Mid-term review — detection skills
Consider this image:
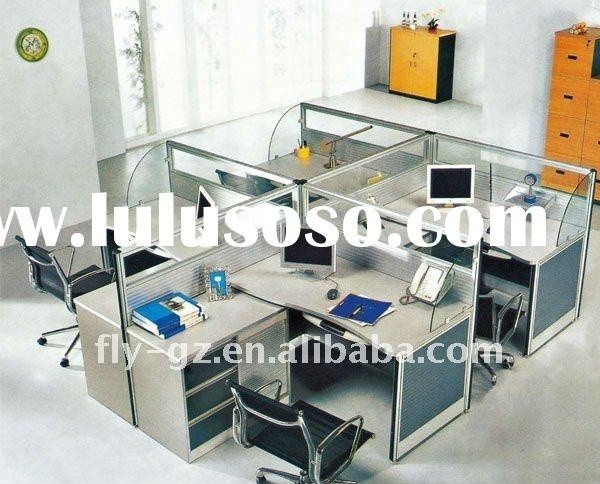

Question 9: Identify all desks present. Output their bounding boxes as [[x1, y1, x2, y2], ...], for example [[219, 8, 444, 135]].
[[72, 102, 596, 464]]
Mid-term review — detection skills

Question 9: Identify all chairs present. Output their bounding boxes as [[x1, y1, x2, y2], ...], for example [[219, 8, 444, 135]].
[[14, 233, 116, 365], [223, 378, 374, 483]]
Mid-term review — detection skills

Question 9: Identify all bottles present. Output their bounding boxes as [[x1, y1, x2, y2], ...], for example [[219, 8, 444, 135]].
[[402, 11, 419, 29]]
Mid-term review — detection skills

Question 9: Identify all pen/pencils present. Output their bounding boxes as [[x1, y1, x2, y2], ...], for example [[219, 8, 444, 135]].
[[349, 306, 363, 317], [297, 138, 306, 148]]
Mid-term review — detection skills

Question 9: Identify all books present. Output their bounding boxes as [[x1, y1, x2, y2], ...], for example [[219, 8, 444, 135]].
[[131, 290, 208, 340]]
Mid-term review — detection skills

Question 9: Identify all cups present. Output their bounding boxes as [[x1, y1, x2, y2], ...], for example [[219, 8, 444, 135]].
[[295, 146, 312, 159]]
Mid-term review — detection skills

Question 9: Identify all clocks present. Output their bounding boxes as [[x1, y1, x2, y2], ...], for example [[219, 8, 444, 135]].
[[16, 27, 48, 62]]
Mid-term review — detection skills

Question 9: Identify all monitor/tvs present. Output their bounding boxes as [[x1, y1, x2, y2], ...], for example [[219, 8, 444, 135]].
[[426, 164, 475, 213], [195, 184, 216, 231], [279, 221, 337, 281]]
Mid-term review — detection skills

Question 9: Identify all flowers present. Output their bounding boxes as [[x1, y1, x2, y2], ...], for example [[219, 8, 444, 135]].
[[422, 8, 447, 25]]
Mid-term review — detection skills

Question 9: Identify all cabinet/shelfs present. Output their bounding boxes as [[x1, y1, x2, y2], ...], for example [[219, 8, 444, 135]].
[[539, 25, 600, 204], [388, 23, 456, 104]]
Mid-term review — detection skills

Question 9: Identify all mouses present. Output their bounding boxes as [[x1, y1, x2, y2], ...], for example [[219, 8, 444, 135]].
[[327, 289, 338, 300]]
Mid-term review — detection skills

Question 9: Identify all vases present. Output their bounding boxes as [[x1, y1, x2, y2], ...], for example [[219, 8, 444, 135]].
[[429, 25, 438, 33]]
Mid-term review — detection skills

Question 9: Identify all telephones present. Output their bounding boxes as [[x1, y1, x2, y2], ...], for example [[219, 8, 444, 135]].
[[409, 262, 448, 306]]
